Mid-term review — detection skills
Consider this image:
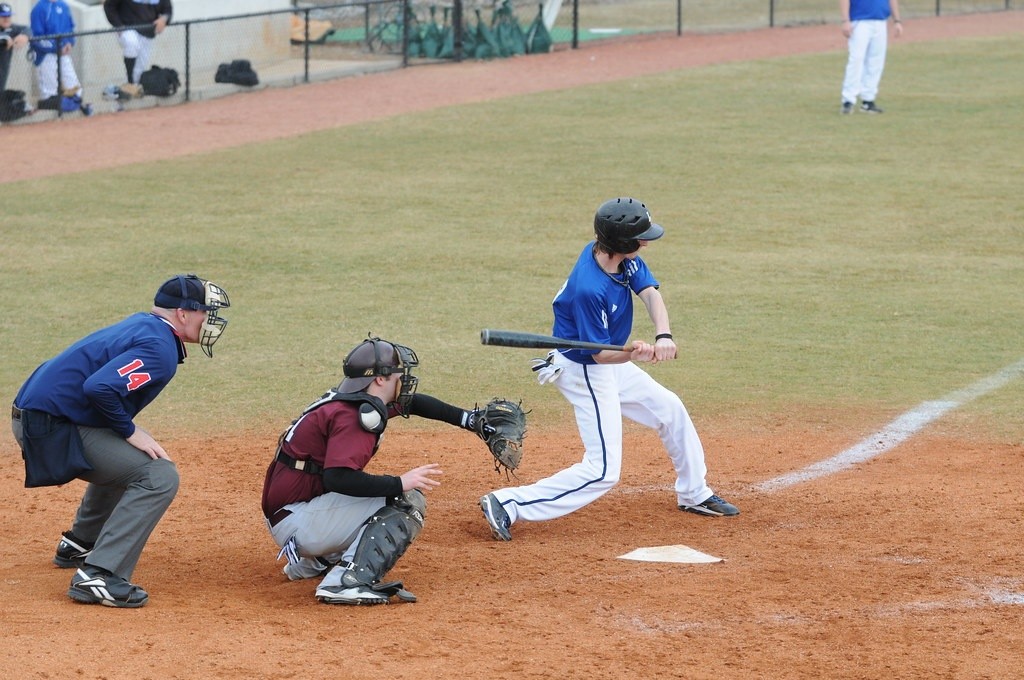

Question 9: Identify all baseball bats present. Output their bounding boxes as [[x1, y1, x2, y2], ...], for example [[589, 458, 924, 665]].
[[480, 328, 679, 359]]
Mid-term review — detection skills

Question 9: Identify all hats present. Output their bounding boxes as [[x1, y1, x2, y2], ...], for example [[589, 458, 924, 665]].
[[0, 3, 12, 16]]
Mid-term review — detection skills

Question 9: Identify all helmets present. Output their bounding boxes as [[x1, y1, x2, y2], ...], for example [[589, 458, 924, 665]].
[[593, 196, 664, 254], [154, 273, 205, 310], [336, 332, 400, 394]]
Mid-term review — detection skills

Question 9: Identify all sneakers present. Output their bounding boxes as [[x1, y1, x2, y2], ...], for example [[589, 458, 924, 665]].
[[68, 564, 149, 608], [52, 530, 94, 569], [677, 494, 740, 518], [314, 586, 390, 606], [840, 102, 854, 114], [859, 101, 882, 114], [477, 494, 514, 543], [283, 562, 300, 582]]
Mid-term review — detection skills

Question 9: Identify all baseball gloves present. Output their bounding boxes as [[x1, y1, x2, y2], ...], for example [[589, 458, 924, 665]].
[[474, 400, 526, 471]]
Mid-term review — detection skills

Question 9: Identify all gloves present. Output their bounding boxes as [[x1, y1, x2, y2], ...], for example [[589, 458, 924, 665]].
[[530, 354, 563, 385]]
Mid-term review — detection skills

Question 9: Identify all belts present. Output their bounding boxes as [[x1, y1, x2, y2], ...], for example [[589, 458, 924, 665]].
[[269, 510, 292, 527], [11, 404, 21, 418]]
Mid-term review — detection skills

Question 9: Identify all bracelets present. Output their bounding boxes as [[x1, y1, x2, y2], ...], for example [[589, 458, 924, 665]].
[[894, 19, 902, 23], [656, 333, 672, 341]]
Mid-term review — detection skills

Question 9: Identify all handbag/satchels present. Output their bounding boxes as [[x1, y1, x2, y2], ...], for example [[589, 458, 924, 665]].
[[140, 66, 180, 96], [214, 59, 259, 87]]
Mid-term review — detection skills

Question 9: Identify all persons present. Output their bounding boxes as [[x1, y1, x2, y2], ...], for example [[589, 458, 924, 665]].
[[0, 4, 33, 92], [27, 1, 83, 102], [104, 0, 172, 84], [12, 274, 230, 606], [262, 335, 528, 605], [480, 199, 740, 541], [839, 0, 904, 114]]
